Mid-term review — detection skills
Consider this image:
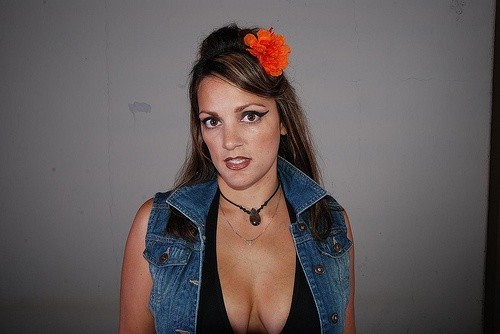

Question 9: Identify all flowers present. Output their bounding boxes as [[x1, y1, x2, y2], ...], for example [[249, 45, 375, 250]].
[[244, 27, 290, 77]]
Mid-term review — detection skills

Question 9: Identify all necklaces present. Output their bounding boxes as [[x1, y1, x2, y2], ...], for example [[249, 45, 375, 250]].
[[217, 179, 281, 227], [216, 189, 283, 248]]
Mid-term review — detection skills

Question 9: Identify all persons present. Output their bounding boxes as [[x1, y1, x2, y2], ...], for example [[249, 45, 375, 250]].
[[117, 23, 359, 334]]
